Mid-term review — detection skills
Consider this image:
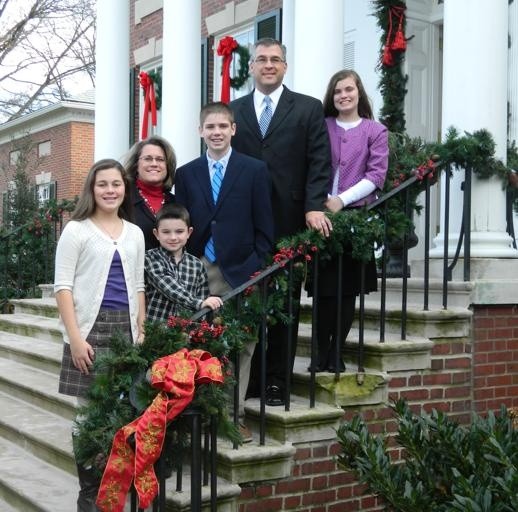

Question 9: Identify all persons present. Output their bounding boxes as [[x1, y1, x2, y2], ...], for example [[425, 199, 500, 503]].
[[173, 101, 276, 444], [54, 157, 148, 511], [303, 69, 391, 374], [119, 135, 184, 253], [227, 37, 336, 409], [144, 204, 226, 479]]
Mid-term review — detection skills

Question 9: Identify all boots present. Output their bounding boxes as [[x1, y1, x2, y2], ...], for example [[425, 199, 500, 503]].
[[72, 430, 102, 512]]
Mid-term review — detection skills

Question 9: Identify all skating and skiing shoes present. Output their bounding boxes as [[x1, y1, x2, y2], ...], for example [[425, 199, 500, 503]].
[[239, 421, 253, 443]]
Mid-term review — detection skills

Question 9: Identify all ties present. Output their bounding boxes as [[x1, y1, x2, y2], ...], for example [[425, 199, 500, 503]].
[[205, 161, 224, 263], [259, 95, 273, 138]]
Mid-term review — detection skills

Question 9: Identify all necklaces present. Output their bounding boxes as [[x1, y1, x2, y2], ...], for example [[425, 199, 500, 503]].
[[95, 215, 120, 240], [138, 190, 166, 218]]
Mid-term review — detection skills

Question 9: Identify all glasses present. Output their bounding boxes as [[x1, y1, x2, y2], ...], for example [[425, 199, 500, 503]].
[[137, 155, 167, 162], [253, 56, 285, 64]]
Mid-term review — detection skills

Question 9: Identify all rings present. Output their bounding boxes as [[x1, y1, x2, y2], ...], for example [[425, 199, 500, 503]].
[[321, 221, 326, 225]]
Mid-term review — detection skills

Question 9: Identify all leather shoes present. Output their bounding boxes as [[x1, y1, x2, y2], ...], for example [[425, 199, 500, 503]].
[[308, 355, 346, 372], [263, 382, 287, 405]]
